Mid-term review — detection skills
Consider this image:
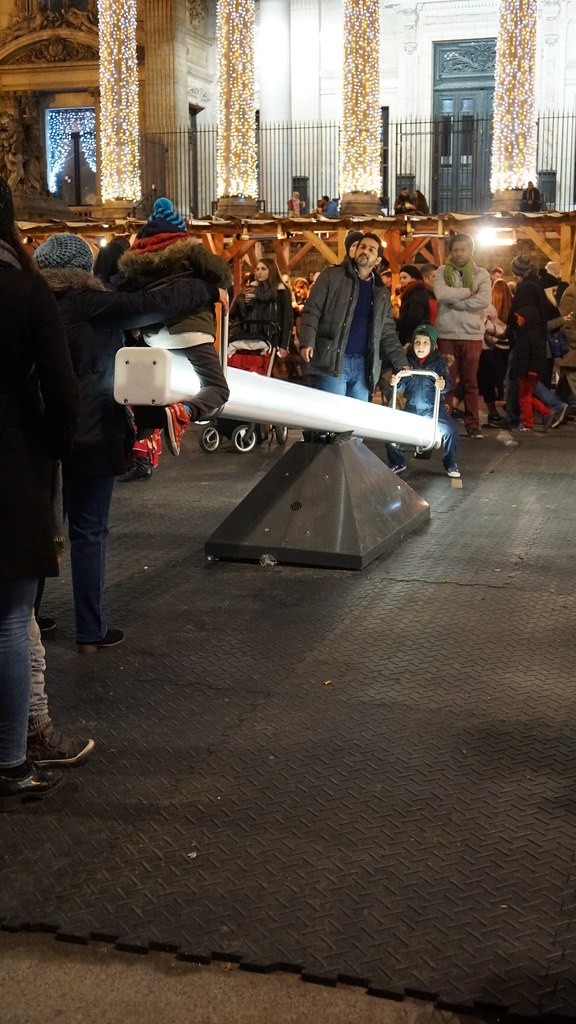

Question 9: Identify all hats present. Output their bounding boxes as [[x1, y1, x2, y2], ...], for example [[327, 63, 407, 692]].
[[344, 229, 365, 253], [33, 233, 93, 272], [148, 197, 186, 231], [413, 320, 437, 346], [137, 221, 184, 238], [399, 264, 423, 279], [511, 254, 532, 278], [545, 262, 562, 279]]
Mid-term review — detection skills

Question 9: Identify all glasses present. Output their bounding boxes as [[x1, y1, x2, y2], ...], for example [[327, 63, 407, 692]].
[[381, 271, 391, 278]]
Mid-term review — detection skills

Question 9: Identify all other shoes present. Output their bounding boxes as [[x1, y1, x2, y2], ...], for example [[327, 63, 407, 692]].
[[551, 403, 569, 428], [512, 424, 534, 432], [488, 415, 504, 424], [496, 429, 518, 446], [543, 412, 554, 431], [468, 428, 483, 438], [450, 409, 464, 418]]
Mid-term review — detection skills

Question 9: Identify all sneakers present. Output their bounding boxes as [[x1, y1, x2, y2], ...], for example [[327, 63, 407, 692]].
[[444, 463, 461, 477], [26, 720, 94, 767], [389, 464, 406, 473], [165, 403, 190, 456]]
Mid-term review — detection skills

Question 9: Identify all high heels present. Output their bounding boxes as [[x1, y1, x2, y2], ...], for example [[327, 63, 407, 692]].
[[76, 629, 125, 653], [35, 617, 56, 631]]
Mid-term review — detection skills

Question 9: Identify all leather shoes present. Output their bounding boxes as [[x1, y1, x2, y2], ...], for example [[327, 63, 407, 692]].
[[0, 760, 65, 813], [113, 458, 151, 483]]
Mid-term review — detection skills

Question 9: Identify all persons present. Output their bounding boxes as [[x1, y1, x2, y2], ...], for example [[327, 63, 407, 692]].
[[520, 181, 541, 212], [394, 187, 429, 215], [296, 228, 576, 476], [320, 196, 339, 217], [288, 191, 307, 215], [231, 258, 321, 381], [0, 181, 234, 810]]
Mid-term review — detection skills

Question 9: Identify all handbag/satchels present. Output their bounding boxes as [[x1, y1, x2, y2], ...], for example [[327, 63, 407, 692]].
[[548, 331, 570, 357]]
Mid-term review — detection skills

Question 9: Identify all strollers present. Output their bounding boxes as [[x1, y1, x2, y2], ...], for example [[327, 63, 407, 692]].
[[200, 318, 289, 454]]
